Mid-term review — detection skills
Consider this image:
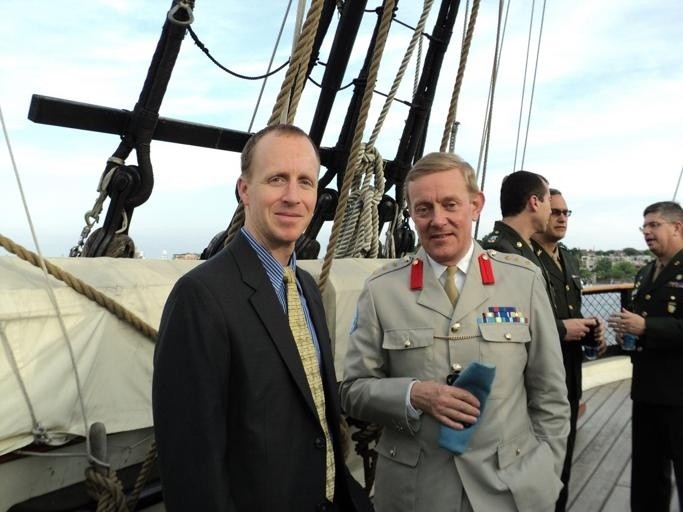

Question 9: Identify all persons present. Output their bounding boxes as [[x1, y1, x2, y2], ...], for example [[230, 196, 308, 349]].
[[526, 186, 609, 510], [476, 166, 576, 510], [336, 152, 574, 511], [147, 121, 374, 511], [604, 201, 683, 511]]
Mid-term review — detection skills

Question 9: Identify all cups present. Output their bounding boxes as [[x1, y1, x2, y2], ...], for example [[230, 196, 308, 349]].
[[583, 317, 601, 360]]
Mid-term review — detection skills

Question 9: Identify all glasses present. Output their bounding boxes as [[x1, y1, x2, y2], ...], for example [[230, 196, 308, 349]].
[[639, 221, 672, 230], [551, 208, 573, 218]]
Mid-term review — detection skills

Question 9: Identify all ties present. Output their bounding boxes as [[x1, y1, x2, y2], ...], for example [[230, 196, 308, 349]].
[[552, 254, 562, 269], [281, 265, 336, 504], [444, 265, 460, 310], [652, 261, 661, 284]]
